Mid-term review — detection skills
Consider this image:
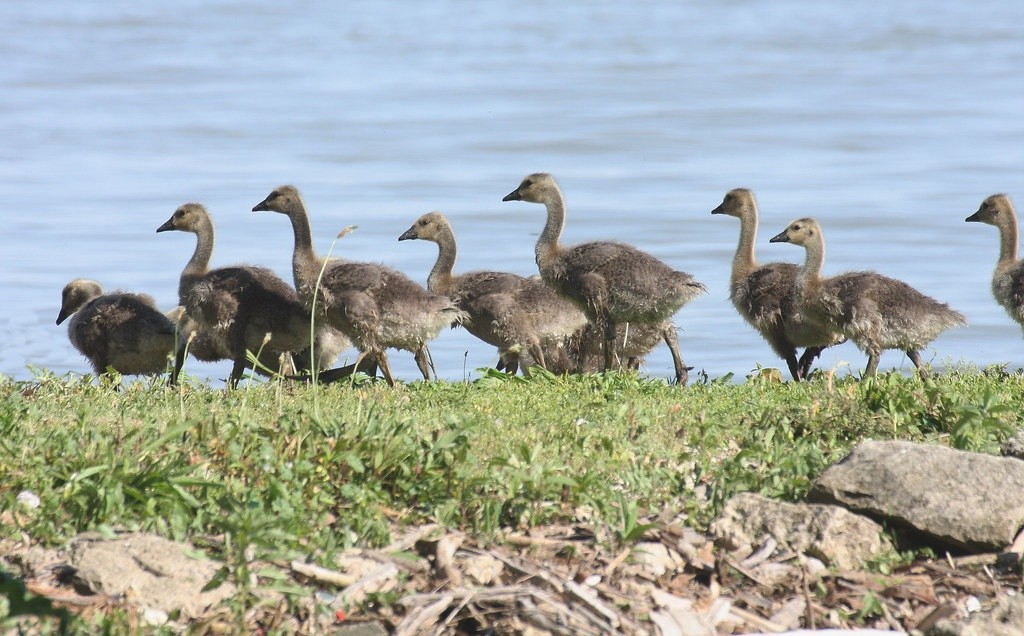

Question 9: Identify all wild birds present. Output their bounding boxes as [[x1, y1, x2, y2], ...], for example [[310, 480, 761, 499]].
[[397, 212, 593, 373], [769, 218, 968, 387], [251, 185, 470, 385], [54, 279, 190, 390], [710, 187, 847, 382], [963, 193, 1024, 327], [519, 275, 647, 379], [161, 303, 351, 380], [503, 171, 710, 387], [156, 204, 326, 390]]
